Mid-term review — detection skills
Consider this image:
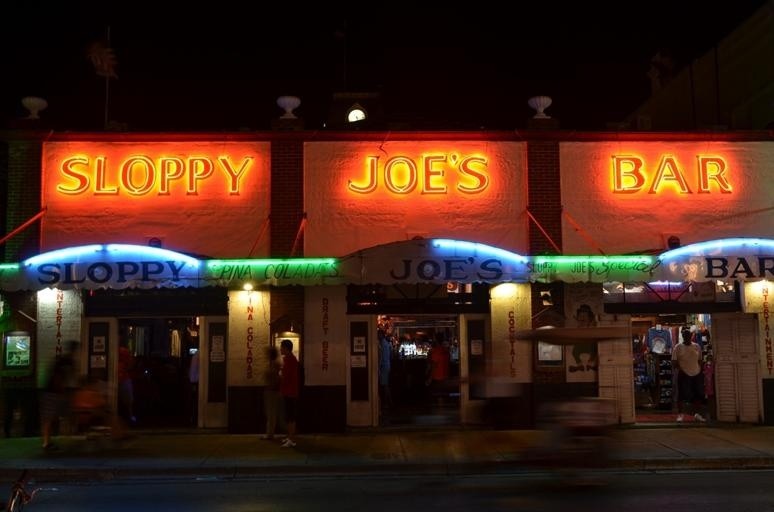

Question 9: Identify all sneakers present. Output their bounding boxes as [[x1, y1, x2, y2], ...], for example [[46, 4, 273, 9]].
[[677, 412, 685, 422], [694, 413, 707, 422], [282, 437, 289, 443], [280, 440, 296, 448]]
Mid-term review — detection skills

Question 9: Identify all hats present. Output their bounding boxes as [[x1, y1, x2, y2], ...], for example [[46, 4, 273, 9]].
[[578, 304, 592, 313]]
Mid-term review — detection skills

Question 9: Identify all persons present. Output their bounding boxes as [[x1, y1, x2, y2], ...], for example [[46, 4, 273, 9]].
[[71, 373, 127, 444], [672, 326, 708, 423], [279, 340, 300, 449], [118, 337, 137, 424], [262, 344, 281, 441], [376, 329, 396, 409], [186, 345, 200, 427], [427, 336, 450, 406], [37, 338, 82, 446]]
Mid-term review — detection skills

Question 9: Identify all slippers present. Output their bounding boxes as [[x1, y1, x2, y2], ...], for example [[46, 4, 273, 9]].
[[41, 441, 58, 451]]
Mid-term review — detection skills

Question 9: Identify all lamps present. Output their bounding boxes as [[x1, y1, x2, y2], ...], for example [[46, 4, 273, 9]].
[[344, 101, 369, 126]]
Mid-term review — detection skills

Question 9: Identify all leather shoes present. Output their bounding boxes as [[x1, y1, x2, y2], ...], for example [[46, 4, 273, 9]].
[[260, 436, 272, 439]]
